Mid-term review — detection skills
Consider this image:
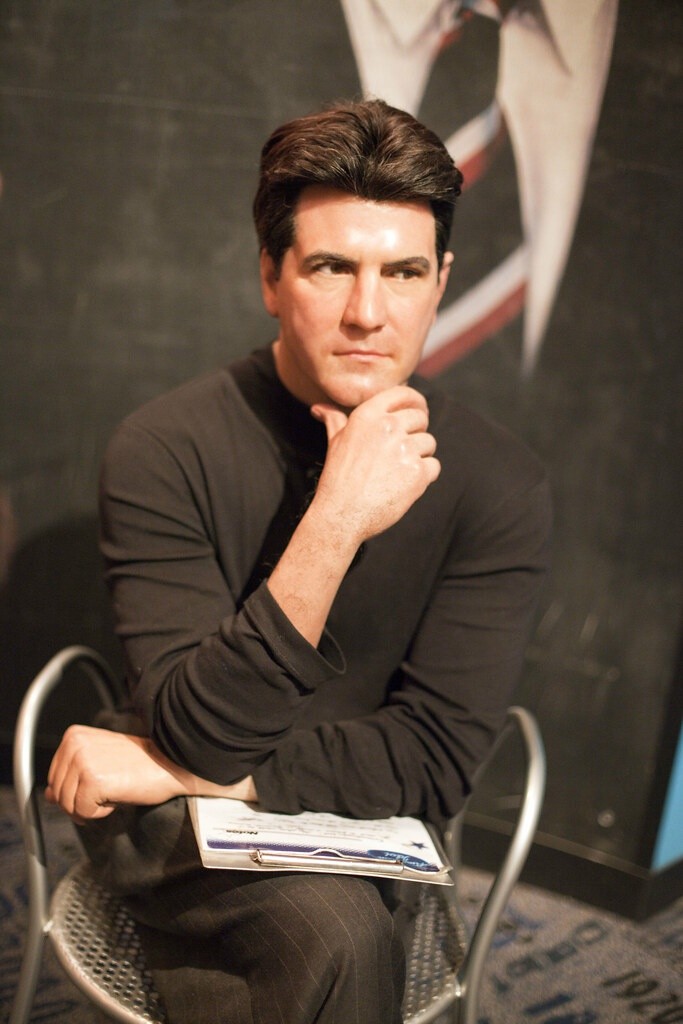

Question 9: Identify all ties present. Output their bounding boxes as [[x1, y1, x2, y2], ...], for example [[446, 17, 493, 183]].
[[412, 0, 526, 422]]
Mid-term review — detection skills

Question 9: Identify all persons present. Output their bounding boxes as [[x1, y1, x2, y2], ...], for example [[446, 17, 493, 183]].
[[183, 0, 682, 843], [43, 103, 549, 1024]]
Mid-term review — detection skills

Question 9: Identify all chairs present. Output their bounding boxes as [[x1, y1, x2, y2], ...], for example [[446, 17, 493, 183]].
[[11, 645, 547, 1024]]
